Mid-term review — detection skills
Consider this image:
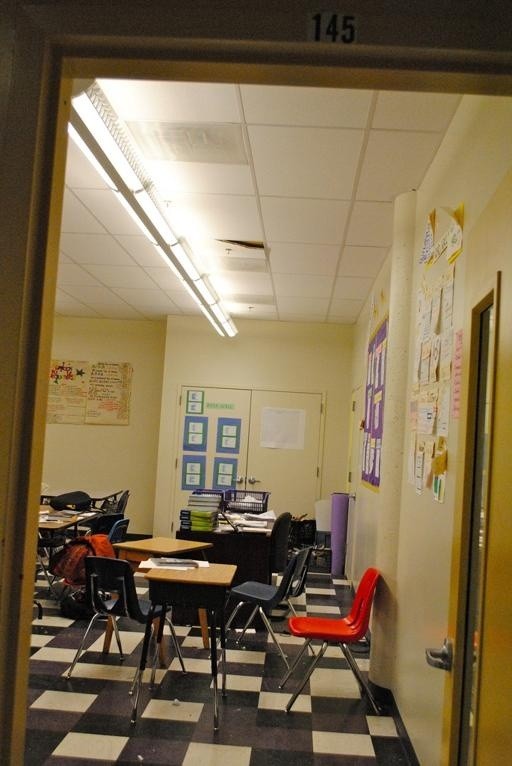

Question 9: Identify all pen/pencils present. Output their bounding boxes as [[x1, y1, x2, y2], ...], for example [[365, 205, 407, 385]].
[[46, 520, 58, 521]]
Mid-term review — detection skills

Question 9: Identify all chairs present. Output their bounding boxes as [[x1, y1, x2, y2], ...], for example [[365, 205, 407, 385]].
[[38, 489, 130, 618], [270, 511, 292, 572], [65, 556, 187, 695], [208, 545, 382, 716]]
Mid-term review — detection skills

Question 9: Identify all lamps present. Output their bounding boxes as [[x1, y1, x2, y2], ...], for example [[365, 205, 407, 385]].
[[67, 90, 238, 339]]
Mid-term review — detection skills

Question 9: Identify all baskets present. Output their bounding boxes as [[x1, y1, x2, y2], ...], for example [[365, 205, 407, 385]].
[[223, 488, 272, 515]]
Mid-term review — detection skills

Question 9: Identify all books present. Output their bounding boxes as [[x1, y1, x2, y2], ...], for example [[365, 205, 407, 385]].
[[179, 488, 268, 532], [46, 513, 72, 522], [151, 557, 198, 569]]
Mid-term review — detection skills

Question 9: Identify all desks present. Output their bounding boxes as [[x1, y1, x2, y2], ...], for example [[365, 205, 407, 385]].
[[171, 529, 272, 627], [111, 536, 239, 732]]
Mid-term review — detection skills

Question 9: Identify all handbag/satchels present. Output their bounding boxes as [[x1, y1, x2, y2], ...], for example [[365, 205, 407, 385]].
[[49, 490, 93, 512]]
